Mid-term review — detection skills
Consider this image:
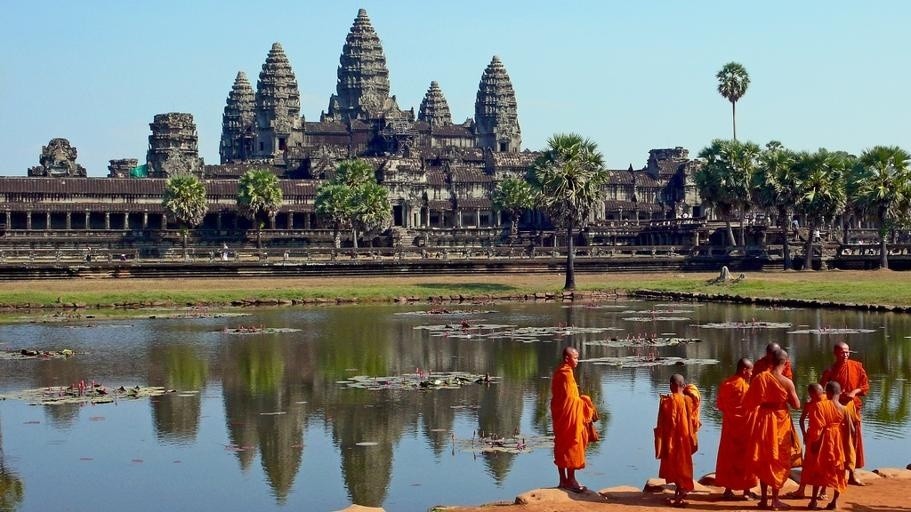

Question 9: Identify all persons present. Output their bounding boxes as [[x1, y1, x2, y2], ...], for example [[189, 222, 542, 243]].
[[858, 239, 863, 255], [814, 228, 821, 242], [550, 346, 600, 494], [653, 342, 870, 510]]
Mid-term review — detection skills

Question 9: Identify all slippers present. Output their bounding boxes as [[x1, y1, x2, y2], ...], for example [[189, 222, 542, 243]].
[[667, 496, 688, 507], [783, 490, 804, 498], [808, 494, 838, 509], [758, 499, 791, 510], [720, 491, 755, 500], [849, 479, 866, 486], [563, 484, 585, 493]]
